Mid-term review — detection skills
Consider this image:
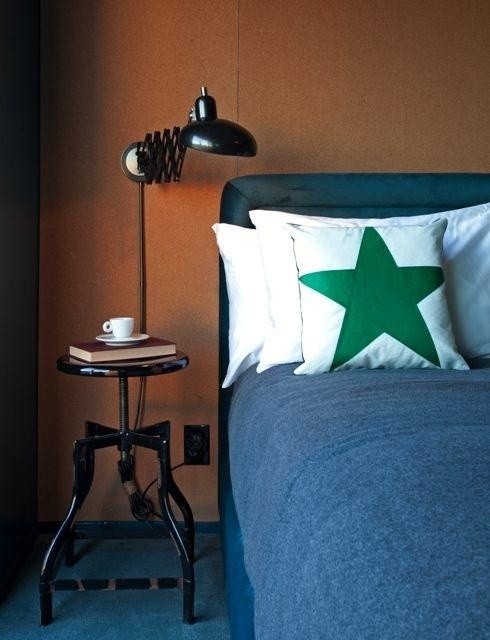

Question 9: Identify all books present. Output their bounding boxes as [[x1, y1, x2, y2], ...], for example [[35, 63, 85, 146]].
[[66, 336, 177, 364]]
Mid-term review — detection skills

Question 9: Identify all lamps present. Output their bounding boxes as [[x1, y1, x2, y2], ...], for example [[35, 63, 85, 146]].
[[115, 84, 258, 523]]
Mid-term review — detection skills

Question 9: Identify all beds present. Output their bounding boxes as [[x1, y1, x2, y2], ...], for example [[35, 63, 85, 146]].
[[218, 171, 490, 640]]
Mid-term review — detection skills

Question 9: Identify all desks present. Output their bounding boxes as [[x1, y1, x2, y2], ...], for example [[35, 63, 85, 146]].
[[36, 352, 199, 627]]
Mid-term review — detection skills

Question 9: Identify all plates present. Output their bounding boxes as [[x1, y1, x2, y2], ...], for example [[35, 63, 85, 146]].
[[96, 332, 150, 347]]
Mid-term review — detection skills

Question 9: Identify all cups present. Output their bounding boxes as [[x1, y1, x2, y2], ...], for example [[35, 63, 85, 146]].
[[102, 316, 135, 339]]
